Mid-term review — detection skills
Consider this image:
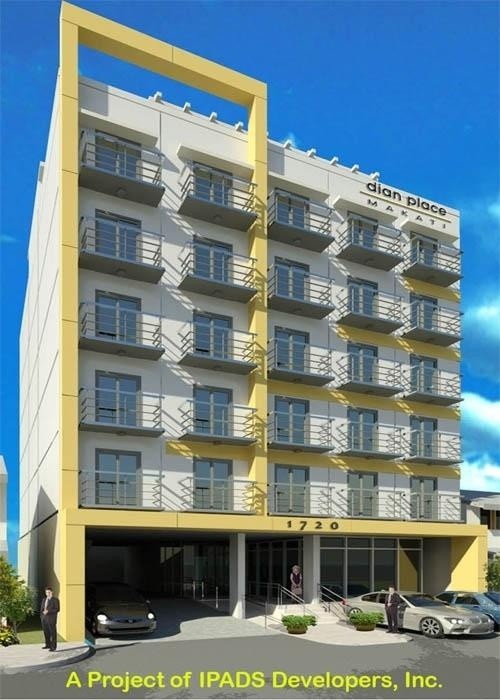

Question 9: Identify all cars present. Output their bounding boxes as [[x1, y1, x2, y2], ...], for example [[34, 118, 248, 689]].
[[342, 591, 500, 638], [85, 584, 158, 638]]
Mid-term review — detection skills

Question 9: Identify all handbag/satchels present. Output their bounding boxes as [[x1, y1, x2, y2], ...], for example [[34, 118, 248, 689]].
[[291, 585, 302, 595]]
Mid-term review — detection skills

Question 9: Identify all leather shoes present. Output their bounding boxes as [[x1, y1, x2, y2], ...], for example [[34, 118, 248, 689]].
[[42, 647, 56, 651]]
